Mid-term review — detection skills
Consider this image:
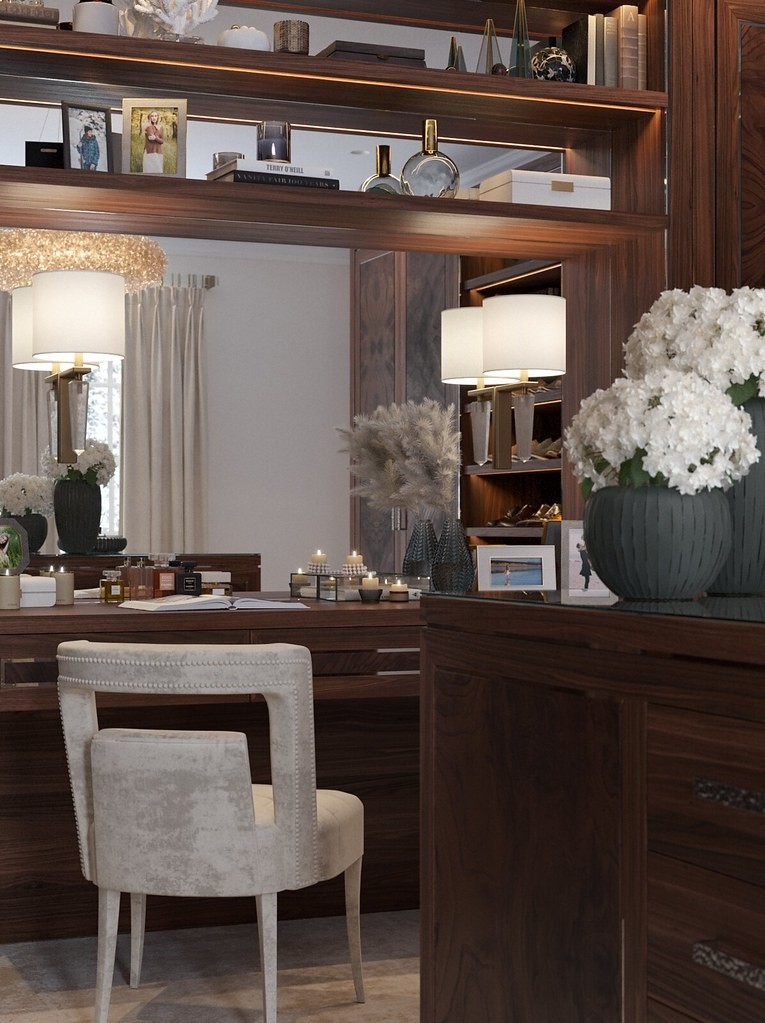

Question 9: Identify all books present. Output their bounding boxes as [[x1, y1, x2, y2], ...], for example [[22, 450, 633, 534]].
[[561, 5, 647, 90], [205, 159, 340, 190], [119, 595, 311, 611], [0, 1, 59, 30]]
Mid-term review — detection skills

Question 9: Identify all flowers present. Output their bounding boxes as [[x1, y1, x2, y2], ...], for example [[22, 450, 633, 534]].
[[563, 367, 761, 497], [0, 472, 54, 518], [621, 284, 765, 406], [0, 437, 117, 519]]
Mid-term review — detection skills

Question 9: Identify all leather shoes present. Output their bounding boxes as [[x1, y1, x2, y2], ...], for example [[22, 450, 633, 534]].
[[524, 503, 561, 524], [515, 504, 551, 526], [486, 505, 523, 527], [499, 504, 539, 527]]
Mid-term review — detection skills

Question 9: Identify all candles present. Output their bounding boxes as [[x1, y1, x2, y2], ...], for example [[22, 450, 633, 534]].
[[391, 583, 408, 591], [362, 578, 379, 589], [51, 571, 74, 605], [312, 554, 327, 564], [292, 574, 308, 583], [347, 555, 363, 564], [43, 572, 51, 577], [0, 576, 21, 610]]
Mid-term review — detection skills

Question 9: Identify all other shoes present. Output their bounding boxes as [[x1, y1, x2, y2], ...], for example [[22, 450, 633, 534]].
[[513, 377, 561, 394], [487, 437, 561, 460]]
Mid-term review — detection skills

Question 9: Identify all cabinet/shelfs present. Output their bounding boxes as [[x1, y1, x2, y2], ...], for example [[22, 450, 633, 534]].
[[420, 597, 765, 1023], [457, 260, 571, 604], [0, 0, 670, 254]]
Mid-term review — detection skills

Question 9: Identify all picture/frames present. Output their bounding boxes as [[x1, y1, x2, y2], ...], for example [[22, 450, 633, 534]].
[[0, 516, 30, 576], [475, 545, 557, 591], [118, 98, 187, 178], [62, 100, 114, 172], [560, 519, 618, 606]]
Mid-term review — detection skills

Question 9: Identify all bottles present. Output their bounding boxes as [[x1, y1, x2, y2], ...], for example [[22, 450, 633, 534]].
[[116, 557, 136, 600], [100, 571, 124, 602], [130, 557, 153, 600], [359, 145, 401, 195], [400, 119, 460, 199], [177, 562, 201, 596], [147, 553, 179, 598]]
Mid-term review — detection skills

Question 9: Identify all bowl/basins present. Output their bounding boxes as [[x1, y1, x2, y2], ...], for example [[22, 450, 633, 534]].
[[218, 29, 271, 51]]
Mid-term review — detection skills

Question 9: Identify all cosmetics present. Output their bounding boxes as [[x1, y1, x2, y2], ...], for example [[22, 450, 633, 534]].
[[360, 118, 460, 199], [213, 152, 244, 169], [99, 554, 201, 602], [256, 121, 292, 163]]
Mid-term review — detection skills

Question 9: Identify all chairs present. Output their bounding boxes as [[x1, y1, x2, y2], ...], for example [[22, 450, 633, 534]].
[[56, 640, 365, 1023]]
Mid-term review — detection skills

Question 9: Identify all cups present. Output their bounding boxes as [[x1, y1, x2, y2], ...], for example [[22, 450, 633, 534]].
[[257, 120, 291, 163], [73, 2, 119, 36], [213, 152, 245, 170]]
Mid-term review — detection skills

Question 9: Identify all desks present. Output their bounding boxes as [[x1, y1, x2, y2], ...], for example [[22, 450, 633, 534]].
[[0, 590, 422, 943]]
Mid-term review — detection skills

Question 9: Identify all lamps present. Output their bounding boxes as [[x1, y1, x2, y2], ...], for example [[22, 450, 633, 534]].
[[11, 269, 125, 464], [441, 295, 567, 467]]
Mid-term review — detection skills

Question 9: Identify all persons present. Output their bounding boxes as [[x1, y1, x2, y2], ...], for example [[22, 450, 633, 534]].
[[505, 565, 510, 585], [77, 126, 100, 170], [0, 534, 10, 561], [576, 536, 595, 590], [143, 111, 164, 173]]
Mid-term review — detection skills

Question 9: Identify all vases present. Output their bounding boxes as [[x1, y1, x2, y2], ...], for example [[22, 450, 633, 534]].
[[9, 514, 48, 553], [703, 396, 765, 622], [53, 478, 101, 553], [403, 521, 438, 577], [431, 520, 474, 596], [585, 485, 731, 617]]
